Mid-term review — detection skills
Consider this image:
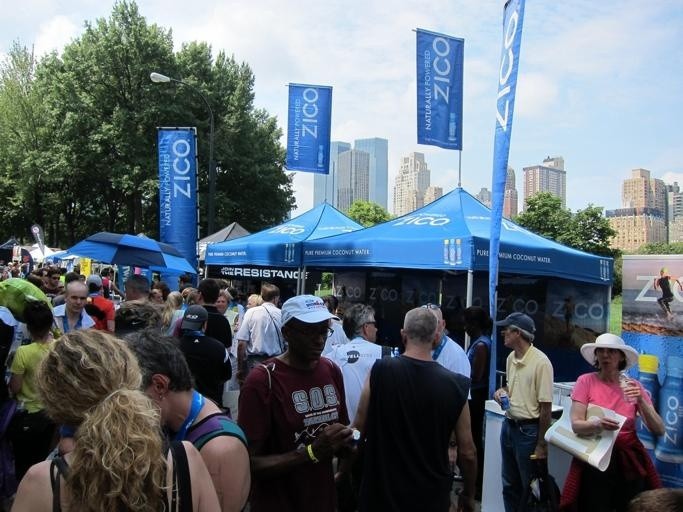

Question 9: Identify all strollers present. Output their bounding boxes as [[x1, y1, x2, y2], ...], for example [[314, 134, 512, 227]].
[[0, 399, 17, 509]]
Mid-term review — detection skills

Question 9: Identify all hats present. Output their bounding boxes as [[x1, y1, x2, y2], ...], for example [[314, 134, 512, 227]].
[[496, 312, 536, 333], [580, 333, 639, 370], [281, 295, 340, 327], [87, 275, 102, 287], [181, 305, 208, 331]]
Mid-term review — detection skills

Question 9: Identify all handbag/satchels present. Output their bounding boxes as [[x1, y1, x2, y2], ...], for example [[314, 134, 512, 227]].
[[527, 459, 561, 512]]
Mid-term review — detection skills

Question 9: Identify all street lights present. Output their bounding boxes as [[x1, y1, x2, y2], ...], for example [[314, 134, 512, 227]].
[[148, 66, 216, 241]]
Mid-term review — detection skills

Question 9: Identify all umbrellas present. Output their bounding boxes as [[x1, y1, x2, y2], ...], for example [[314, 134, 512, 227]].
[[66, 231, 197, 275]]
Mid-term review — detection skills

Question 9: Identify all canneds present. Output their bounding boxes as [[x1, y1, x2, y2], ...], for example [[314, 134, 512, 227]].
[[500, 395, 509, 411]]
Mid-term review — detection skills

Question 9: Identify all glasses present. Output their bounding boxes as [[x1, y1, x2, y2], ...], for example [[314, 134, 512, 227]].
[[291, 325, 334, 337], [421, 304, 438, 309]]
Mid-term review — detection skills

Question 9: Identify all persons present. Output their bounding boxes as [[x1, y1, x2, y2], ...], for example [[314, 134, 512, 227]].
[[630, 487, 683, 512], [494, 312, 555, 512], [559, 332, 665, 512], [654, 269, 683, 320]]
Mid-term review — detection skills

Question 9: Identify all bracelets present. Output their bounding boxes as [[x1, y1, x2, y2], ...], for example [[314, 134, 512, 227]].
[[588, 415, 603, 435]]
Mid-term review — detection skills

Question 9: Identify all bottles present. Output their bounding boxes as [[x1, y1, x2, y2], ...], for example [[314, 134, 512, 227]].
[[500, 396, 510, 411], [393, 347, 399, 356], [631, 354, 662, 450], [617, 370, 637, 405], [657, 356, 682, 463]]
